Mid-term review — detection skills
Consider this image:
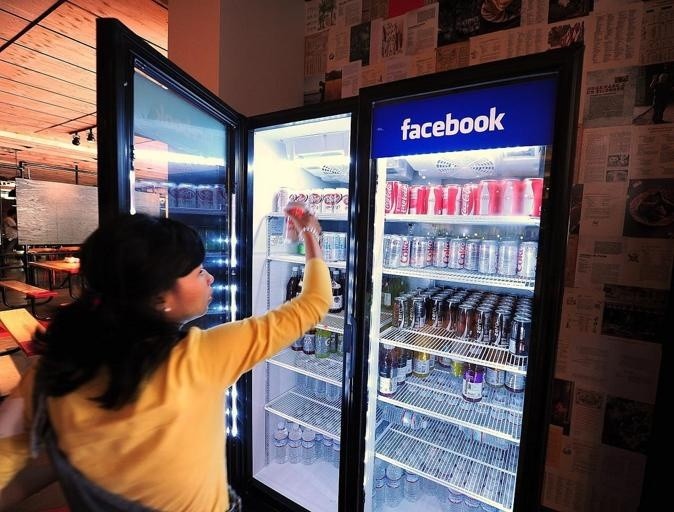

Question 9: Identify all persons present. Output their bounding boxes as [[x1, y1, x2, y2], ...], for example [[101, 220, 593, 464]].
[[3, 206, 18, 251], [0, 208, 333, 512], [650, 73, 671, 124]]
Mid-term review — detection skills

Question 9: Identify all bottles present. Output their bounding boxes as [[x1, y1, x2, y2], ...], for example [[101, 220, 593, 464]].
[[273, 264, 526, 511]]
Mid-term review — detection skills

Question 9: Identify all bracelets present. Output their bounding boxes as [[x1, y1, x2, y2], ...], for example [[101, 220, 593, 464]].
[[300, 227, 319, 242]]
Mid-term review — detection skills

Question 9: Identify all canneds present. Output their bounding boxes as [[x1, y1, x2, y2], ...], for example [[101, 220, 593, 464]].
[[278, 185, 349, 355], [382, 179, 544, 358], [134, 179, 226, 210], [186, 226, 226, 254]]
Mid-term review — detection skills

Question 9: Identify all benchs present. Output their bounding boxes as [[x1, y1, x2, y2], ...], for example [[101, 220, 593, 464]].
[[0, 279, 59, 358]]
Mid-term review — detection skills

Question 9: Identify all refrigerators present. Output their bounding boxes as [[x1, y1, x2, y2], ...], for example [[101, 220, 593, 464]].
[[89, 15, 588, 512]]
